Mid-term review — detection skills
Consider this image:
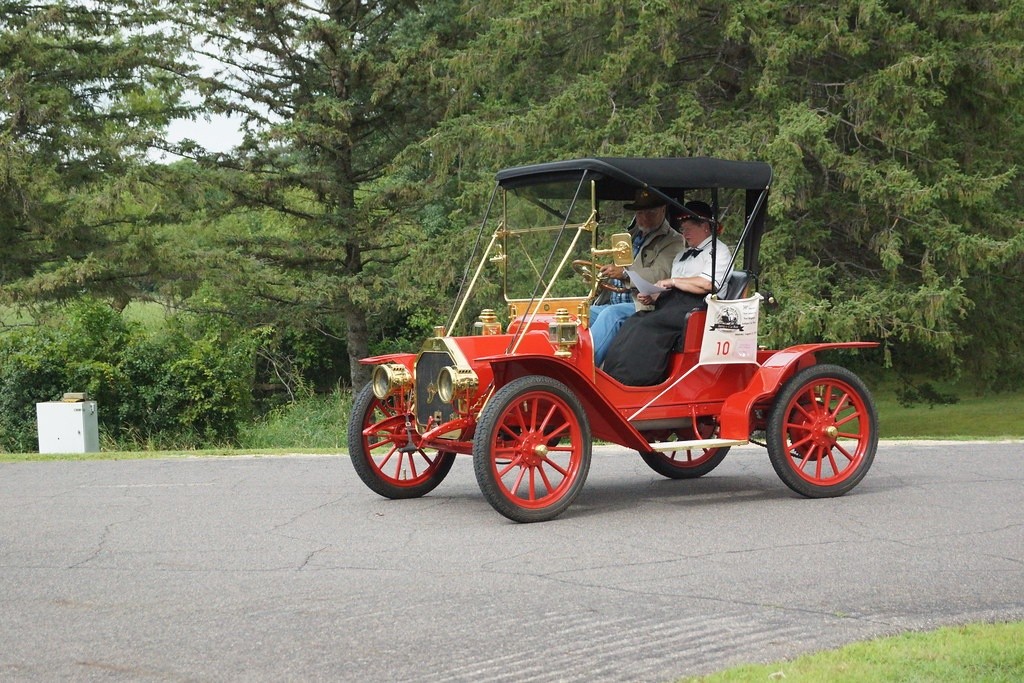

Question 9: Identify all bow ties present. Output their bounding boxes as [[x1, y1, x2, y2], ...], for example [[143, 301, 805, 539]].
[[679, 248, 703, 261]]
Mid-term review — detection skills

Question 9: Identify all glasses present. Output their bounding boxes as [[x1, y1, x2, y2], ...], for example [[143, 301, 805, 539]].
[[635, 207, 663, 216], [679, 223, 701, 232]]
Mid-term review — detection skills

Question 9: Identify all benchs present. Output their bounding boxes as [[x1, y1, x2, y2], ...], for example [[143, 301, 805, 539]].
[[675, 272, 748, 353]]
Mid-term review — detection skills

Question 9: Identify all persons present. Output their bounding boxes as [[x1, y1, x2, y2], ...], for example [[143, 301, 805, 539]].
[[602, 201, 735, 386], [587, 191, 685, 368]]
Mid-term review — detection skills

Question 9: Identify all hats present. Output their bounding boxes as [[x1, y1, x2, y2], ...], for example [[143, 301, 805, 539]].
[[664, 201, 725, 236], [623, 186, 671, 210]]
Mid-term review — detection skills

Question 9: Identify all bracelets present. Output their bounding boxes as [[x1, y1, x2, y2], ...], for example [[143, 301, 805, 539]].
[[622, 270, 628, 280], [672, 277, 676, 287]]
